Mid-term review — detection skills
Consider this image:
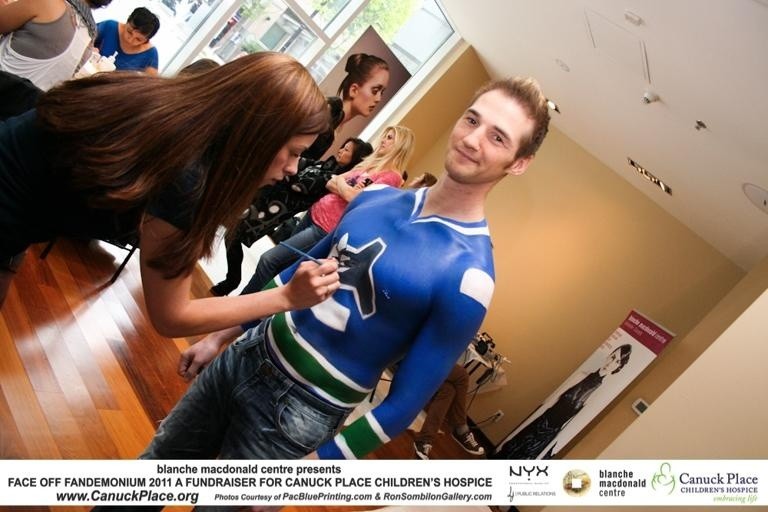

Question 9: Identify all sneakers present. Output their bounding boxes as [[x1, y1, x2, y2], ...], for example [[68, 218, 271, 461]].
[[413, 442, 432, 461], [450, 429, 484, 456]]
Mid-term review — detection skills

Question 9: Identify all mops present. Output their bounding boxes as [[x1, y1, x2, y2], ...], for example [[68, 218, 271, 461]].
[[98, 48, 118, 74]]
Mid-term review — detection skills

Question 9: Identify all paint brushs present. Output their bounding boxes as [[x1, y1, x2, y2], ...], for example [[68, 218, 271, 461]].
[[280, 242, 323, 265]]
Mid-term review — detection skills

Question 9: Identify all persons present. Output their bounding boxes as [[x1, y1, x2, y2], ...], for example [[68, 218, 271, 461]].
[[205, 137, 374, 297], [0, 0, 112, 122], [298, 53, 391, 160], [407, 171, 438, 190], [0, 51, 340, 339], [96, 75, 552, 512], [489, 343, 632, 460], [412, 363, 485, 461], [238, 125, 415, 302], [93, 6, 160, 76]]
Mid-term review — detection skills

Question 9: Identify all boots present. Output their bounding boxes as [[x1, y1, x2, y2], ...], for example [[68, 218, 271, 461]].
[[210, 276, 240, 297]]
